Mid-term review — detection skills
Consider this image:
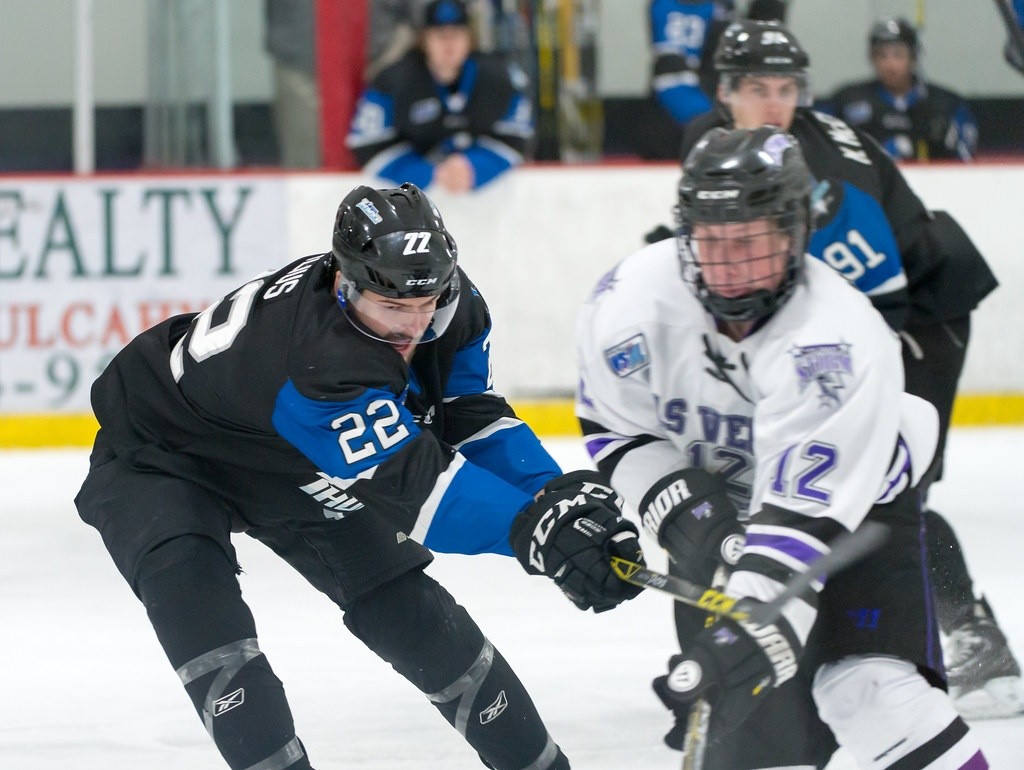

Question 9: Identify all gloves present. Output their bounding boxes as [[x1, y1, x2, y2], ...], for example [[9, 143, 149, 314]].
[[511, 470, 651, 611], [654, 597, 806, 752], [636, 470, 746, 593]]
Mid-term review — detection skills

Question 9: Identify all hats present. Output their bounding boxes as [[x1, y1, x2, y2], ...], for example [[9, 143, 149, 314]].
[[420, 0, 471, 26]]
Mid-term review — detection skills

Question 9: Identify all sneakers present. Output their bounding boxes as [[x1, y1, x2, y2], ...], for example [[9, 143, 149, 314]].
[[939, 595, 1023, 721]]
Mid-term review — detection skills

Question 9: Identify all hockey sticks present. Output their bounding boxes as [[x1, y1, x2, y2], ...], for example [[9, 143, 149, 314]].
[[612, 519, 895, 626], [680, 695, 712, 770]]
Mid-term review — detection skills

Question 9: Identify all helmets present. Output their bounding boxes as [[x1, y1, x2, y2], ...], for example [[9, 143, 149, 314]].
[[866, 17, 919, 63], [675, 125, 815, 324], [716, 19, 816, 107], [336, 181, 461, 344]]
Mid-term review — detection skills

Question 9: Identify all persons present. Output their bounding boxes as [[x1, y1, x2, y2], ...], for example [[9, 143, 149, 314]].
[[676, 18, 1002, 511], [814, 15, 980, 161], [643, 0, 734, 161], [574, 125, 992, 770], [345, 0, 538, 191], [263, 0, 605, 170], [73, 181, 647, 770]]
